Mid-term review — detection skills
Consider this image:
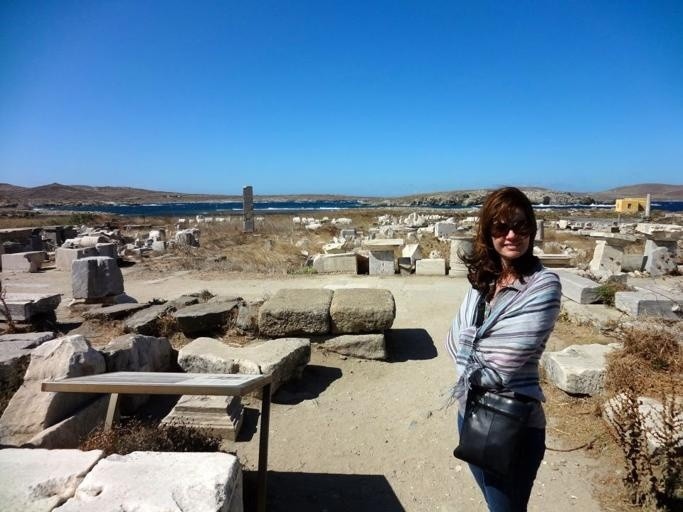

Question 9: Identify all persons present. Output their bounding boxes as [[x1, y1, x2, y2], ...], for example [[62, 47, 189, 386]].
[[611, 222, 620, 233], [446, 187, 561, 512]]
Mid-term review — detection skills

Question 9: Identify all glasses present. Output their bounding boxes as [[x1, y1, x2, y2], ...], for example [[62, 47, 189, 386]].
[[489, 222, 530, 238]]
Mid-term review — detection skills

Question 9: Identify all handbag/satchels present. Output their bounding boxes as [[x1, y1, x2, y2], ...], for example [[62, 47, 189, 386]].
[[453, 385, 530, 475]]
